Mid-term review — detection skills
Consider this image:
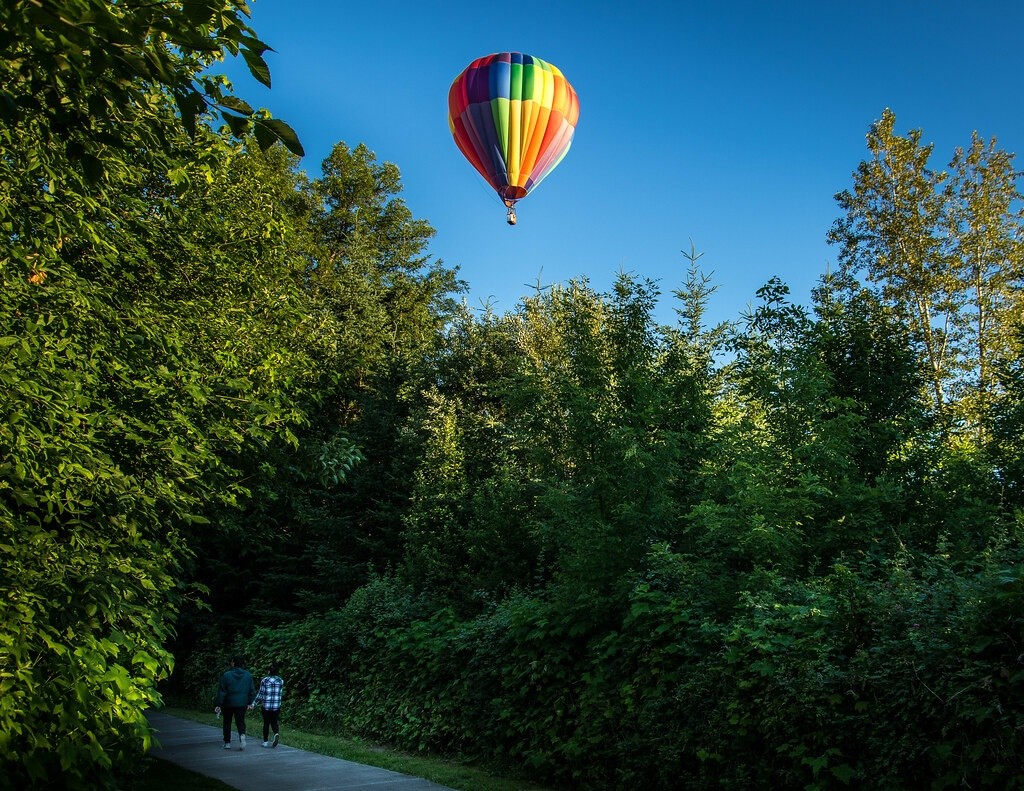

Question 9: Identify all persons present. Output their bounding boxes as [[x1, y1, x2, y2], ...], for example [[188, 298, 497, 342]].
[[250, 665, 284, 748], [213, 656, 256, 750]]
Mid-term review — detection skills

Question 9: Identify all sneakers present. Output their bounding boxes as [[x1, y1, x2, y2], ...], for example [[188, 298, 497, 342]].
[[240, 734, 246, 749], [273, 733, 280, 747], [262, 742, 268, 747], [223, 743, 231, 748]]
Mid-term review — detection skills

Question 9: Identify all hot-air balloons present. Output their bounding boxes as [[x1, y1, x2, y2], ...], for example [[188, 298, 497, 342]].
[[447, 53, 580, 225]]
